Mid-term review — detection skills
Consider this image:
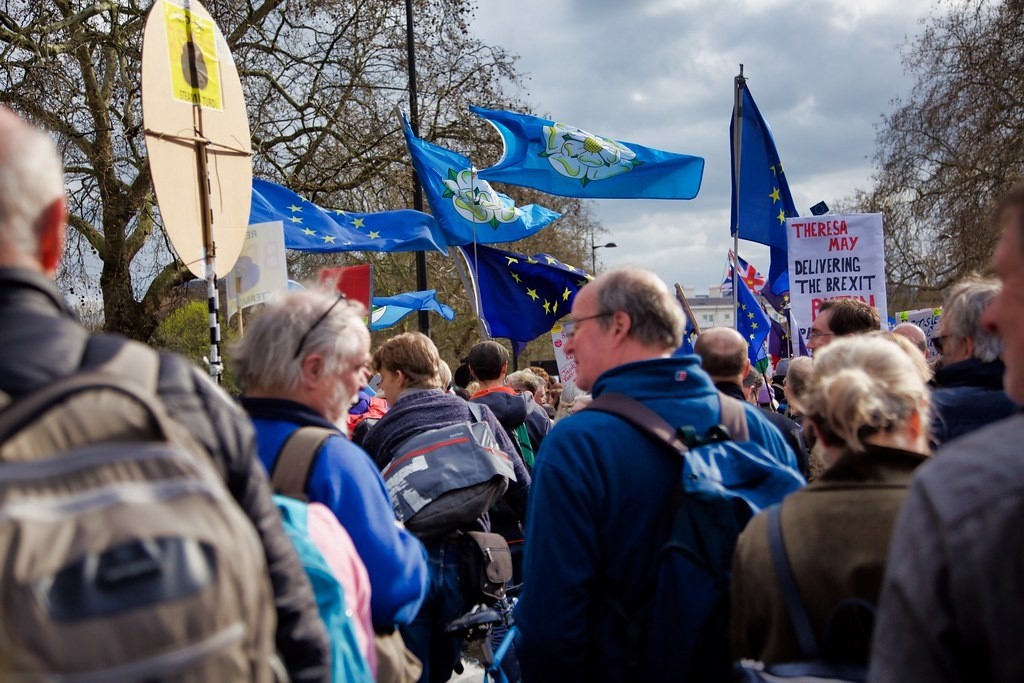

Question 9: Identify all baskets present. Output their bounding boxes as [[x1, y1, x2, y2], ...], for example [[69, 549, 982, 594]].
[[489, 597, 516, 664]]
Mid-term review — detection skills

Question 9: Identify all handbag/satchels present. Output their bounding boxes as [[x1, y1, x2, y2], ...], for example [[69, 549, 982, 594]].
[[382, 420, 517, 530], [466, 531, 515, 602]]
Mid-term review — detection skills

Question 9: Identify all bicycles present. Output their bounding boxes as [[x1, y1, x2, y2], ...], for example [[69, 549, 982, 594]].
[[445, 592, 524, 683]]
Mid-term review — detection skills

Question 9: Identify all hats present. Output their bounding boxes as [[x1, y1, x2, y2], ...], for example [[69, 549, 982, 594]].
[[771, 357, 788, 378], [469, 341, 509, 379], [756, 384, 775, 403]]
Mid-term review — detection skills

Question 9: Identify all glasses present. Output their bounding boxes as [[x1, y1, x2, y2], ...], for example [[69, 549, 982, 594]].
[[561, 310, 612, 337], [294, 290, 347, 359], [806, 330, 837, 340], [932, 333, 954, 354]]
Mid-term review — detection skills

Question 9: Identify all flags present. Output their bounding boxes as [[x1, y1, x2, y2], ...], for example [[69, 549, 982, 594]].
[[721, 84, 809, 366], [458, 242, 595, 341], [470, 105, 705, 200], [674, 289, 696, 345], [249, 179, 449, 257], [403, 128, 564, 246], [370, 290, 455, 330]]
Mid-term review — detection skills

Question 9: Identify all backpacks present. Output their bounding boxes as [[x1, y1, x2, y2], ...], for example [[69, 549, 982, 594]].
[[0, 335, 289, 683], [576, 389, 808, 683], [733, 501, 871, 683]]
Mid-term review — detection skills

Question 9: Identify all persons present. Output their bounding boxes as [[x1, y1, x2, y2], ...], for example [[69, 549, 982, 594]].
[[344, 333, 563, 683], [513, 269, 799, 683], [693, 186, 1024, 683], [0, 106, 331, 683], [228, 288, 432, 638]]
